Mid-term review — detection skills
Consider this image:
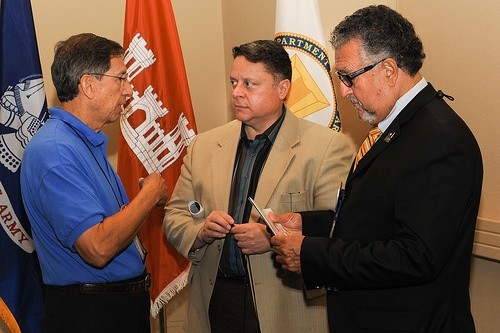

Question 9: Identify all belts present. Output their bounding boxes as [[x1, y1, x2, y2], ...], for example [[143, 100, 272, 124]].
[[60, 272, 152, 292]]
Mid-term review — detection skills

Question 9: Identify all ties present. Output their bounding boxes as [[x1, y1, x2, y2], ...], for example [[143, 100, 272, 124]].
[[353, 128, 383, 172]]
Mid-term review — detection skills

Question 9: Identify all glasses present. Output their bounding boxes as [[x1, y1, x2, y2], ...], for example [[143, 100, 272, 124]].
[[334, 58, 401, 87], [78, 72, 129, 87]]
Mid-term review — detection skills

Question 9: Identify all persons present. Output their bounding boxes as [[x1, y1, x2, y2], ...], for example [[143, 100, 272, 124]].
[[162, 40, 354, 333], [20, 33, 167, 333], [267, 4, 484, 333]]
